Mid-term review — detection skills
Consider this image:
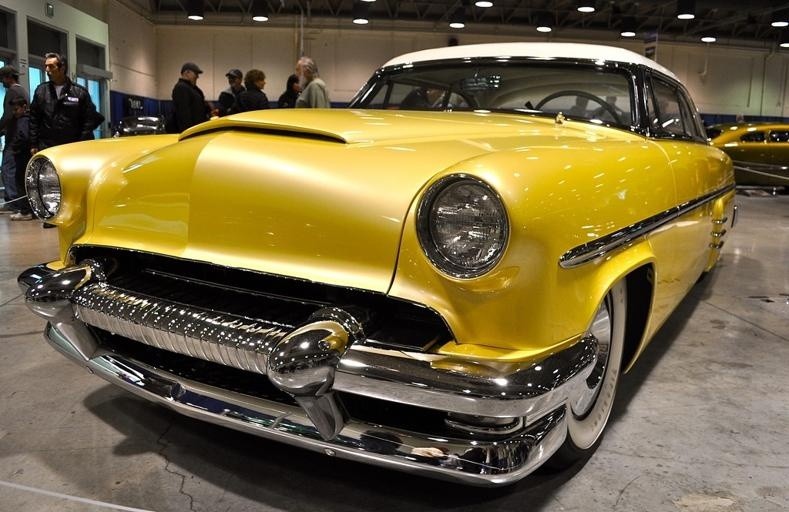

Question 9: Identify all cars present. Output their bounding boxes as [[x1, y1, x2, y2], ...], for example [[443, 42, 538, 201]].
[[16, 40, 740, 491], [110, 116, 165, 136], [700, 119, 789, 190]]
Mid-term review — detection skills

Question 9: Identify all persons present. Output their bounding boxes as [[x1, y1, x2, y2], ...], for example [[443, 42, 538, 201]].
[[0, 64, 31, 211], [9, 97, 34, 220], [219, 69, 246, 117], [294, 56, 330, 108], [399, 86, 445, 110], [171, 62, 212, 132], [236, 69, 270, 111], [29, 53, 96, 228], [278, 74, 302, 108]]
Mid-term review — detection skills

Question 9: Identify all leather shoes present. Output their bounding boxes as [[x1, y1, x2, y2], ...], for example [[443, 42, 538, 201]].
[[42, 222, 56, 228]]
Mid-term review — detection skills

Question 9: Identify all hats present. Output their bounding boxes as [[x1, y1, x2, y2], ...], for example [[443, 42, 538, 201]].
[[180, 62, 203, 75], [225, 68, 242, 80]]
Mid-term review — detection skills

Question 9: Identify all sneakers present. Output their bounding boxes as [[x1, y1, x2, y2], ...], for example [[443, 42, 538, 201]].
[[9, 212, 33, 221]]
